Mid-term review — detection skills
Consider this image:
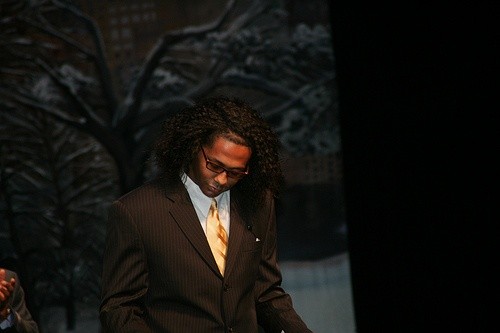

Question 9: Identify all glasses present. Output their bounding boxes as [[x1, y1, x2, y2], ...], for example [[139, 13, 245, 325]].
[[201, 145, 249, 180]]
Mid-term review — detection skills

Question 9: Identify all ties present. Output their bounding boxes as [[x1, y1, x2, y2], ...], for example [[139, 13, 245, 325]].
[[206, 200, 229, 279]]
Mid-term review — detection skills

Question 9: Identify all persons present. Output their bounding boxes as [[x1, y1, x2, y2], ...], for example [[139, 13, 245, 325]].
[[98, 96, 313, 333], [0, 267, 40, 333]]
